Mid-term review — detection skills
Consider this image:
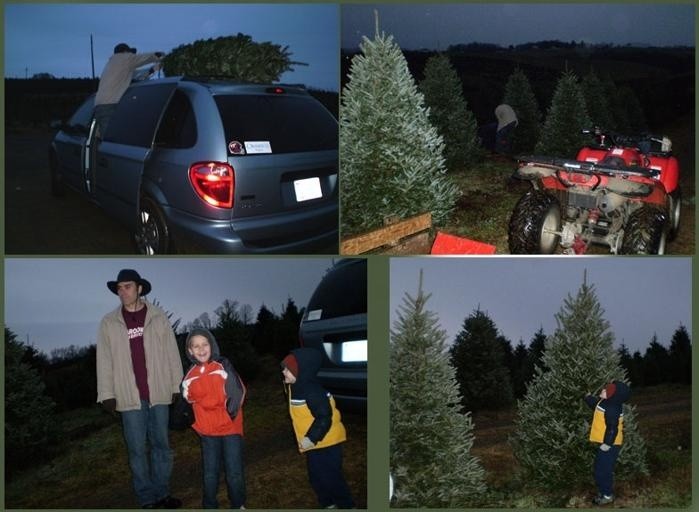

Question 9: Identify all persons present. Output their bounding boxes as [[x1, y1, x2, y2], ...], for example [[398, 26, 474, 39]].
[[281, 347, 355, 509], [179, 327, 247, 508], [92, 43, 165, 141], [581, 380, 631, 506], [494, 103, 519, 156], [95, 269, 185, 509]]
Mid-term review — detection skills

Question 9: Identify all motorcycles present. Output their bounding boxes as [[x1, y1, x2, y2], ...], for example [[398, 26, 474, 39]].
[[502, 127, 684, 255]]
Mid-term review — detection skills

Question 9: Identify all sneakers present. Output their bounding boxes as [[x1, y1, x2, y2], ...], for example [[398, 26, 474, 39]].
[[144, 496, 183, 509], [593, 494, 614, 506]]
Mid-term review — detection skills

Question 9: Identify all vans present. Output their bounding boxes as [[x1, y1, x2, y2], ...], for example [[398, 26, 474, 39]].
[[297, 258, 367, 416], [47, 77, 340, 254]]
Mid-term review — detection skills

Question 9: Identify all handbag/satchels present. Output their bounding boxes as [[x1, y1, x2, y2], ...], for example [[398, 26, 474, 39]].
[[168, 396, 195, 430]]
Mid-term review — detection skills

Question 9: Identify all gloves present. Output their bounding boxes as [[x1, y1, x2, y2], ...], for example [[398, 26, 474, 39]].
[[300, 436, 313, 449], [600, 444, 610, 452], [102, 398, 116, 416]]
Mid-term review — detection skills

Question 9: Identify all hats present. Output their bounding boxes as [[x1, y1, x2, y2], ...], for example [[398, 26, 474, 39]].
[[114, 44, 137, 53], [283, 354, 298, 378], [606, 383, 616, 398], [107, 269, 152, 297]]
